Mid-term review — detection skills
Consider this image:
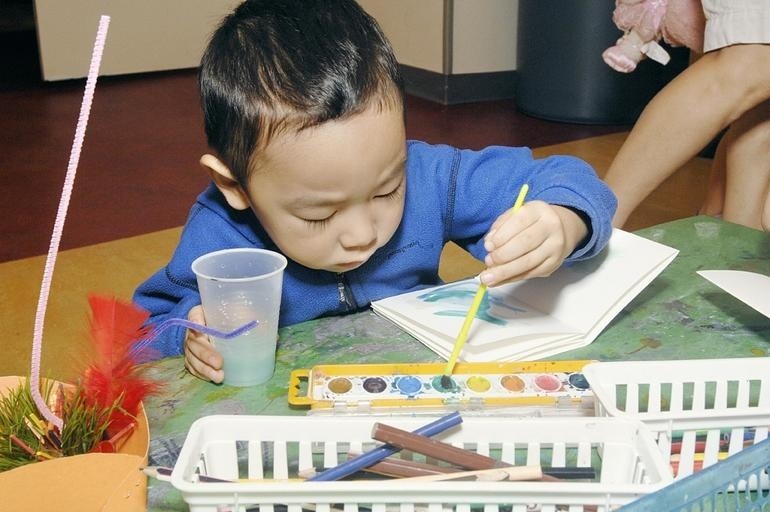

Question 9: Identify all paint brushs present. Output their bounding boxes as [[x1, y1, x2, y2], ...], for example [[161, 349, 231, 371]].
[[441, 183, 529, 388]]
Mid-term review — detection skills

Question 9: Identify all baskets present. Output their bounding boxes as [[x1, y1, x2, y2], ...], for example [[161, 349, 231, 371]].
[[169, 414, 675, 511], [613, 437, 770, 512], [168, 356, 770, 512], [583, 357, 769, 435]]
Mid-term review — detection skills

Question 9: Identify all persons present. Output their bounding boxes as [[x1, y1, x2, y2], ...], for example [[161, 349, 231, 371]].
[[128, 1, 618, 383], [601, 0, 770, 235]]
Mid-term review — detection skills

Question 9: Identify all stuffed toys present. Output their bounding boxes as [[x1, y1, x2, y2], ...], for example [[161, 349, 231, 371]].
[[602, 0, 706, 73]]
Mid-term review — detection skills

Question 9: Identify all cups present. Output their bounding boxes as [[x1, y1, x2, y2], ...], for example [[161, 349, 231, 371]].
[[187, 246, 289, 389]]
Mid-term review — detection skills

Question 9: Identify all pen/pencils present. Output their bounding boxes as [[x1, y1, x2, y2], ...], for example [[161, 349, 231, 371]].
[[656, 427, 770, 479], [305, 411, 595, 483]]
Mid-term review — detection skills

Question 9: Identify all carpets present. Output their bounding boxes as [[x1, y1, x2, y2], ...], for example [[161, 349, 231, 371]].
[[1, 126, 768, 384]]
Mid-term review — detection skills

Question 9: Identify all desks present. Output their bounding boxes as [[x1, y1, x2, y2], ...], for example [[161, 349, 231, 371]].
[[1, 212, 769, 511]]
